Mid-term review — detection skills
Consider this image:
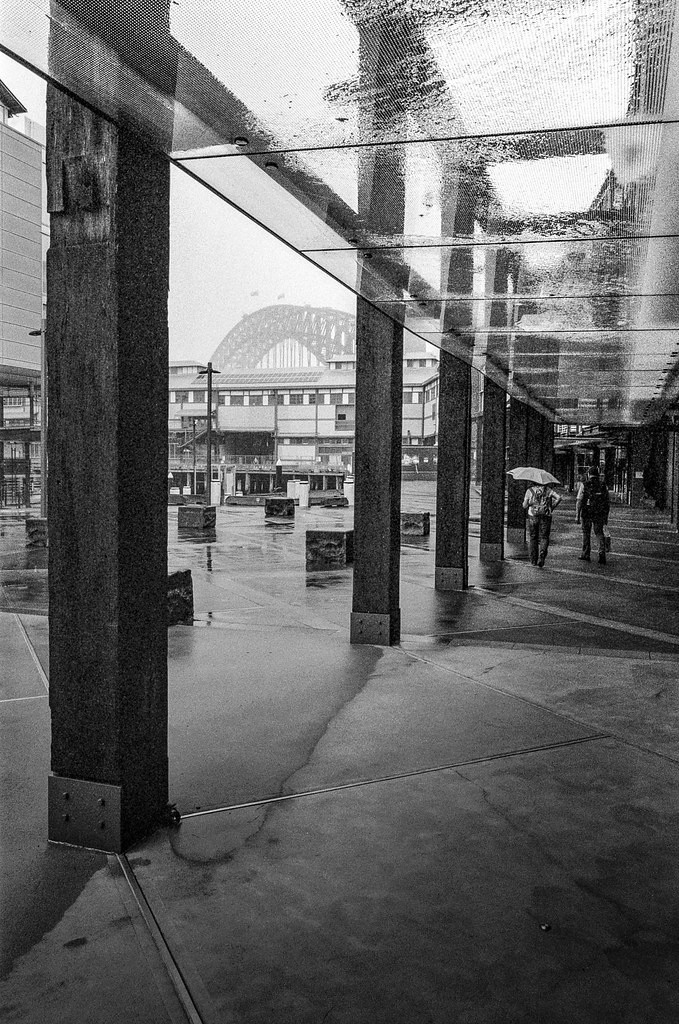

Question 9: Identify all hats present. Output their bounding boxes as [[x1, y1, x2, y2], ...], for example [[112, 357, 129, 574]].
[[586, 467, 597, 473]]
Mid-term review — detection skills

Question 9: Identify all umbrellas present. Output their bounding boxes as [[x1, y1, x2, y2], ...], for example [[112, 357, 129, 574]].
[[506, 466, 561, 485]]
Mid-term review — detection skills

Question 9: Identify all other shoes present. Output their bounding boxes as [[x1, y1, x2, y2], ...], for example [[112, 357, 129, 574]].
[[537, 554, 545, 568], [597, 553, 605, 564], [529, 557, 537, 565], [579, 554, 590, 561]]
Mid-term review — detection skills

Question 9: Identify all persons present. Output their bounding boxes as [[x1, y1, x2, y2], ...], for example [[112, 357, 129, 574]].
[[522, 481, 562, 567], [574, 466, 610, 565]]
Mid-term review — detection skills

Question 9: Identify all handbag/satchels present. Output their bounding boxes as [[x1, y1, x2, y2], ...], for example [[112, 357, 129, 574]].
[[603, 523, 612, 552]]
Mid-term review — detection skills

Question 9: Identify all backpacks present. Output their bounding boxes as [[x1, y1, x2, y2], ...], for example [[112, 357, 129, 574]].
[[587, 481, 609, 518], [529, 486, 549, 516]]
